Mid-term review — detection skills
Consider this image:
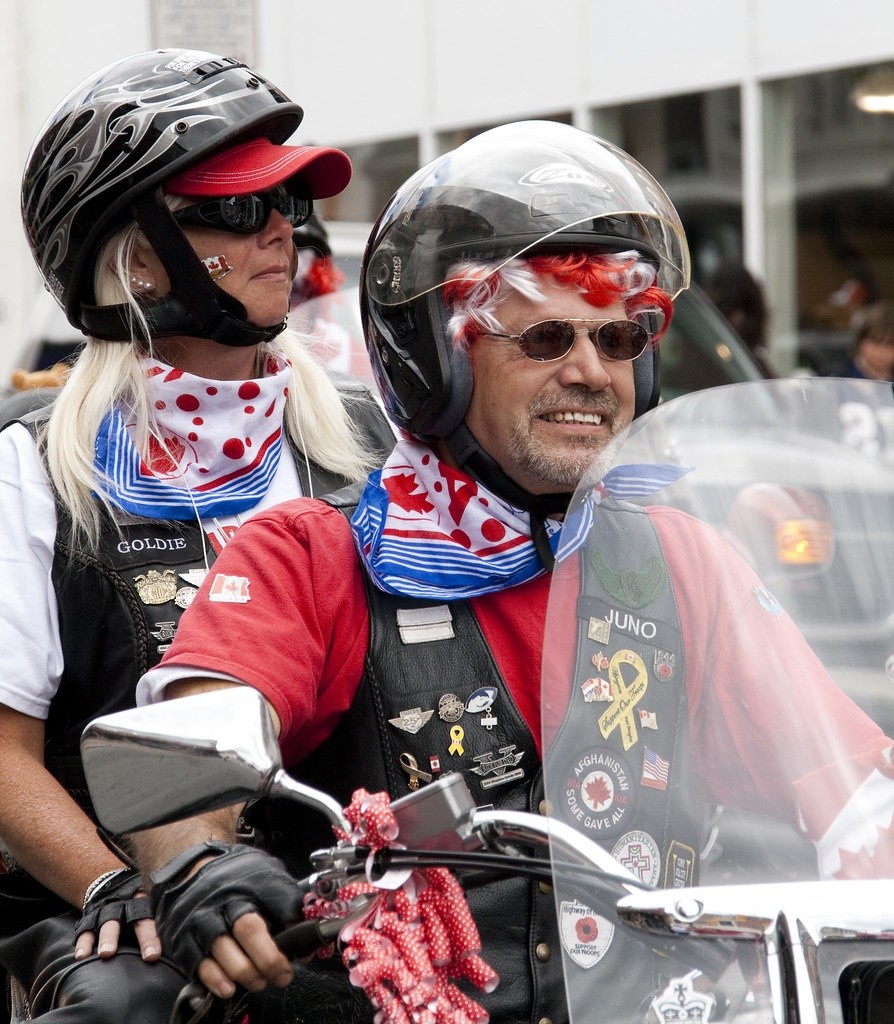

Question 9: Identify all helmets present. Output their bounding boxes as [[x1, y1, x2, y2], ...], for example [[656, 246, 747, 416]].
[[22, 47, 304, 331], [359, 144, 661, 442]]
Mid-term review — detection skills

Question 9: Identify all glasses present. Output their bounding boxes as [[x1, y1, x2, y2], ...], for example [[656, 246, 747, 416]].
[[475, 318, 655, 363], [169, 179, 295, 235]]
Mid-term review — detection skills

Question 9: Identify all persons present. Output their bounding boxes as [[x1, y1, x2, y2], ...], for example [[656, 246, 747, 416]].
[[702, 263, 776, 382], [828, 299, 894, 380], [124, 119, 894, 1023], [0, 40, 411, 1024]]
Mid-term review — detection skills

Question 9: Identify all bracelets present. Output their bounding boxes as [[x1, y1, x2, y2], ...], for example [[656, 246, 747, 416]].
[[82, 866, 131, 910]]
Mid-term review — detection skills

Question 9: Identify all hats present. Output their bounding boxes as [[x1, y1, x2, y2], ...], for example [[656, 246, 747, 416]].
[[165, 140, 350, 195]]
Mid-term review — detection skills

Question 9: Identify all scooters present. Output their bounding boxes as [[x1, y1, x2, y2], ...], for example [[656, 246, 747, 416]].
[[79, 377, 894, 1023]]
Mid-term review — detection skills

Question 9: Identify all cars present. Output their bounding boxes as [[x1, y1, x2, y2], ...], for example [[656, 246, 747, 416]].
[[9, 218, 890, 718]]
